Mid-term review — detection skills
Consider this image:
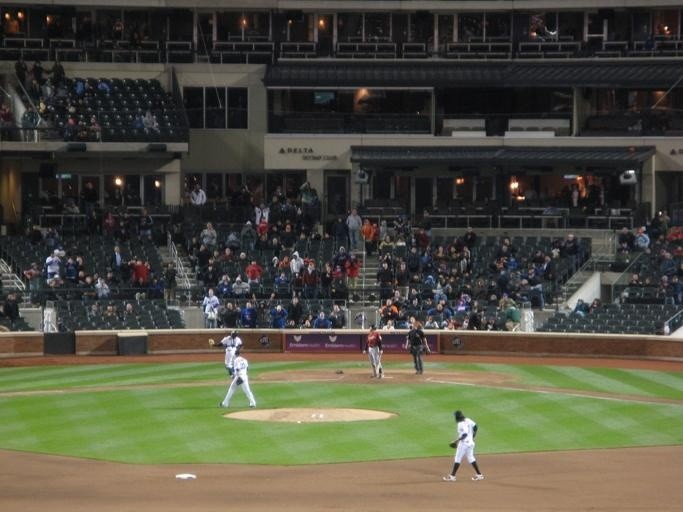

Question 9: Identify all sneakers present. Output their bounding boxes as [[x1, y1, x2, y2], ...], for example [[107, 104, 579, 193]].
[[472, 473, 484, 481], [442, 474, 456, 481]]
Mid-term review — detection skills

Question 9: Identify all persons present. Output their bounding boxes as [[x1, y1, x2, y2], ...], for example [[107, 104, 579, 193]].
[[0, 6, 682, 336], [218, 347, 256, 409], [403, 321, 432, 373], [207, 329, 243, 378], [442, 409, 485, 483], [360, 323, 385, 378]]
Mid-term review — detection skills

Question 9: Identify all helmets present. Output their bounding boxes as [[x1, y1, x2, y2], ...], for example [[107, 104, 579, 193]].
[[230, 331, 238, 337]]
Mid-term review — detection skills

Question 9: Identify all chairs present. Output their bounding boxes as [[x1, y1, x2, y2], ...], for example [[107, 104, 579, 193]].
[[0, 77, 683, 337]]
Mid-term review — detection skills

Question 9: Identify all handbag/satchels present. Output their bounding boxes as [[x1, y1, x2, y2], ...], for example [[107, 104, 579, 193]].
[[207, 306, 215, 320]]
